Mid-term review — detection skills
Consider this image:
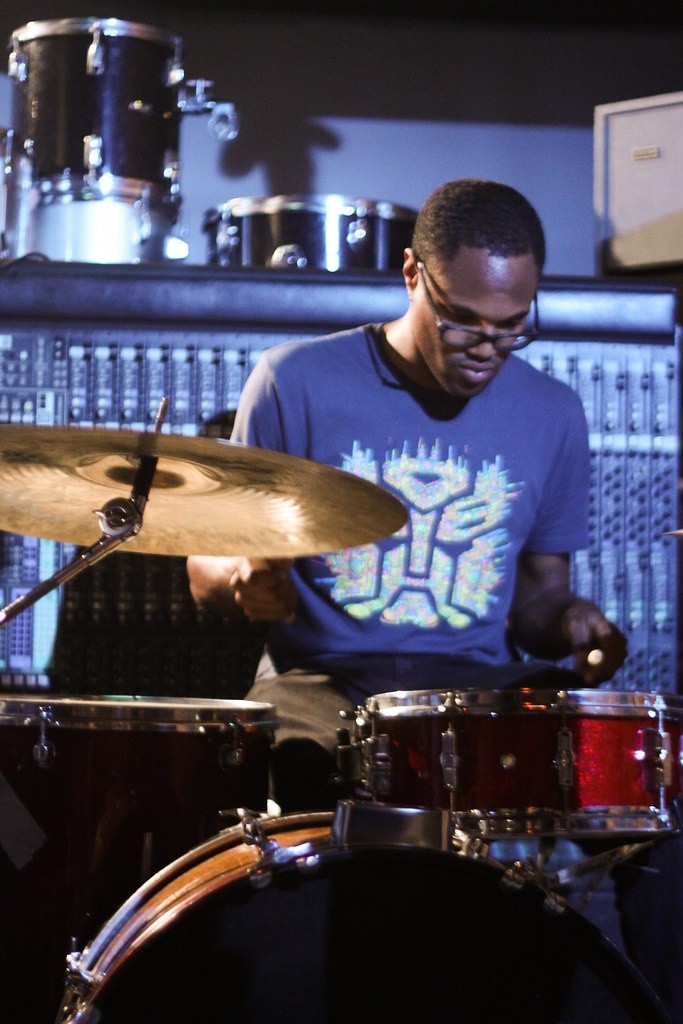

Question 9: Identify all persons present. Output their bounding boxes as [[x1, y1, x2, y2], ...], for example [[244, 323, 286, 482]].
[[185, 178, 627, 819]]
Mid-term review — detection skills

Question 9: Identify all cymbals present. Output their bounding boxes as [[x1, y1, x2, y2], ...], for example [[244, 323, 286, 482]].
[[0, 420, 403, 564]]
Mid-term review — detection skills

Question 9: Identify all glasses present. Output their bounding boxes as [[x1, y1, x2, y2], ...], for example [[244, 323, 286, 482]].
[[415, 256, 540, 352]]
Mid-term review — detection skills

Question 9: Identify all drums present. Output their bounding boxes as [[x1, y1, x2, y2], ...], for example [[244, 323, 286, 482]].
[[198, 191, 418, 277], [3, 16, 188, 204], [58, 809, 674, 1024], [13, 171, 170, 263], [2, 688, 277, 1024], [339, 686, 683, 841]]
